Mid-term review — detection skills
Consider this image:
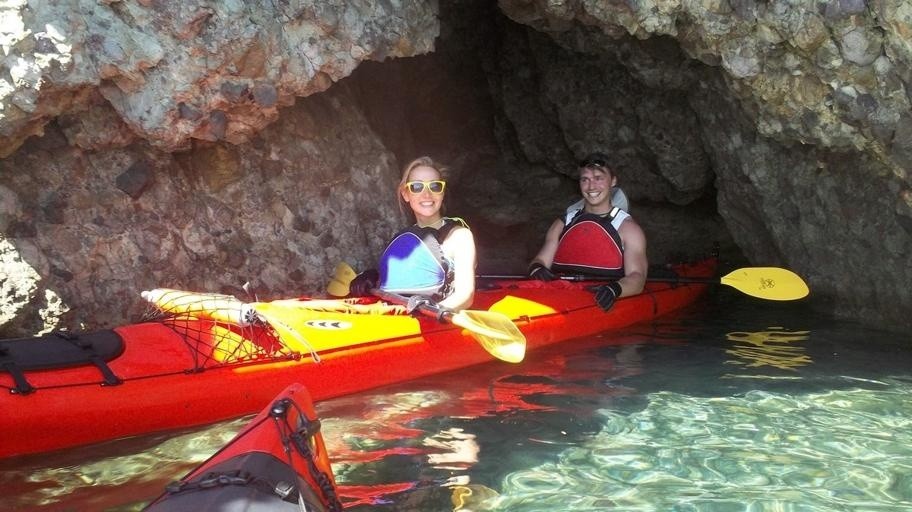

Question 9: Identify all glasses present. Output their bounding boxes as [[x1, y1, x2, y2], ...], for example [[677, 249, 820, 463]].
[[404, 180, 446, 194], [580, 159, 607, 167]]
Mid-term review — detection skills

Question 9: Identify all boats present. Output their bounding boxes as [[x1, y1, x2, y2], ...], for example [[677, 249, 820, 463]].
[[139, 382, 344, 512], [0, 258, 717, 462]]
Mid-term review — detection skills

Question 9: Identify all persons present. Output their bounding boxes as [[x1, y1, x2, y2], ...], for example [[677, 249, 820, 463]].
[[528, 153, 649, 313], [348, 152, 476, 312]]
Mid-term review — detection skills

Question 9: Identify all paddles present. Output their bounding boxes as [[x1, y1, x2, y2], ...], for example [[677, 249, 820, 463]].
[[473, 265, 810, 300], [326, 262, 527, 366]]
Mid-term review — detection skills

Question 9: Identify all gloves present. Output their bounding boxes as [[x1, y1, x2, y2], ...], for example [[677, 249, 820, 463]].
[[587, 282, 622, 311], [405, 294, 438, 314], [527, 263, 556, 282], [349, 268, 378, 298]]
